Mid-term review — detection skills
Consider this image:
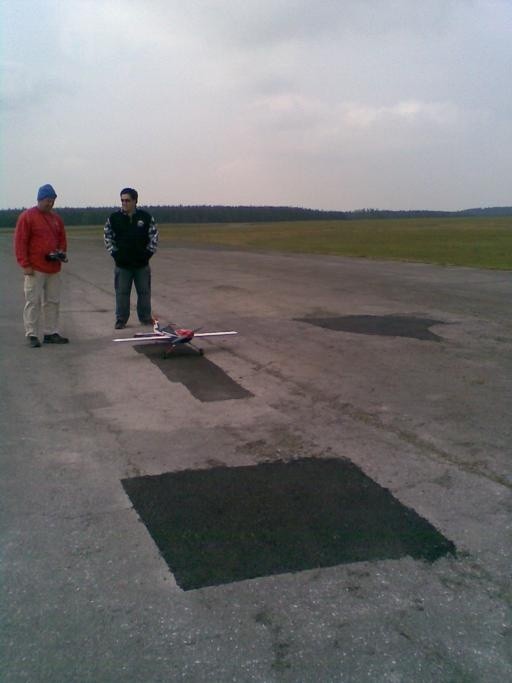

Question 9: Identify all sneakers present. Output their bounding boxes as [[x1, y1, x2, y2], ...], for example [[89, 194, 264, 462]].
[[141, 319, 159, 326], [43, 334, 70, 344], [28, 335, 42, 348], [114, 319, 127, 329]]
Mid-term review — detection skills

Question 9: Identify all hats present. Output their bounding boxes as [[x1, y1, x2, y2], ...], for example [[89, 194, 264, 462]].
[[37, 183, 57, 202]]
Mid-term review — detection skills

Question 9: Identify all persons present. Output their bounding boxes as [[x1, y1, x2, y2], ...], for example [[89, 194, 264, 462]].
[[104, 188, 159, 329], [14, 184, 69, 347]]
